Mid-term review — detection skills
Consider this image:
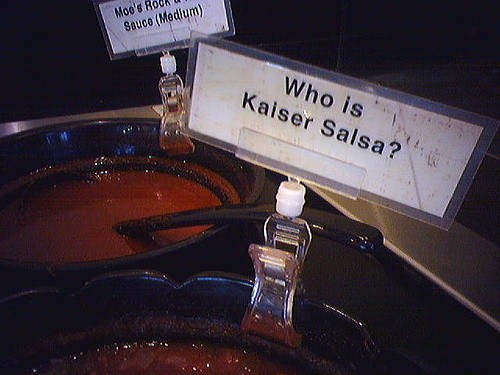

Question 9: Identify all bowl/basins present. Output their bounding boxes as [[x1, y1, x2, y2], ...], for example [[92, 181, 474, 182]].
[[0, 118, 266, 294], [1, 269, 438, 375]]
